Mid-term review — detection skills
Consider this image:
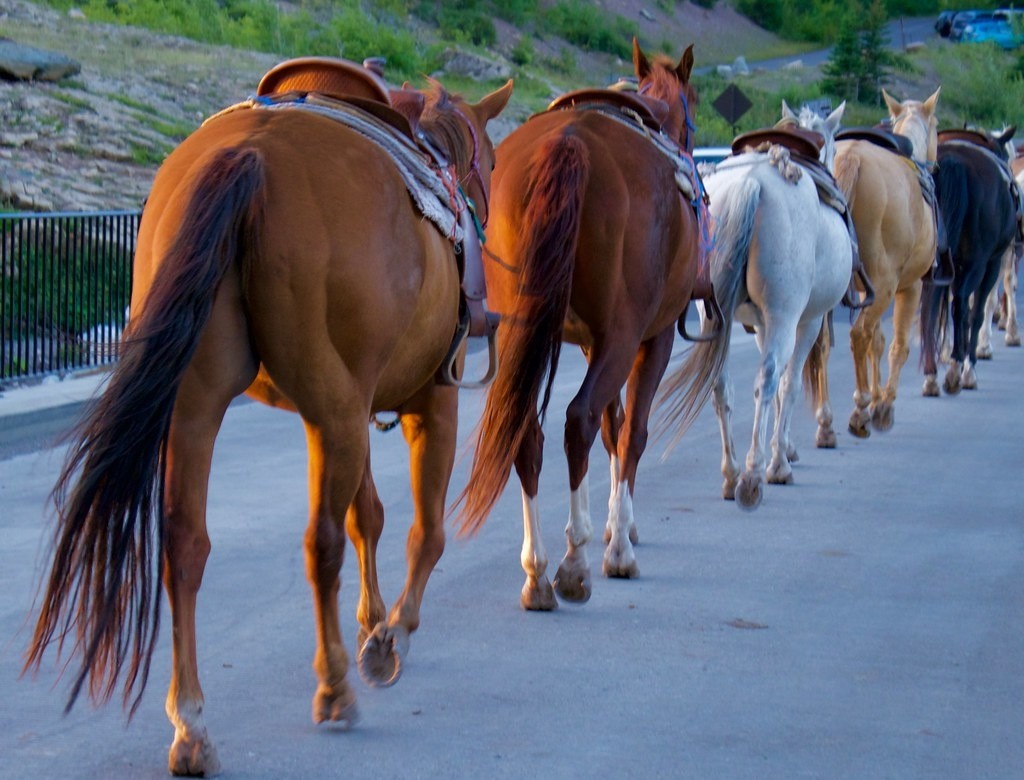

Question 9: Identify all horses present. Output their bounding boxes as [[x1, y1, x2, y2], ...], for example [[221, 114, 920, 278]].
[[912, 122, 1024, 399], [799, 85, 942, 448], [480, 36, 700, 614], [648, 99, 854, 512], [8, 78, 514, 780]]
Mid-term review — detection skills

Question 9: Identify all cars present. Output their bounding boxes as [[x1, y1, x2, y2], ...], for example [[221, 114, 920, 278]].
[[934, 8, 1024, 50]]
